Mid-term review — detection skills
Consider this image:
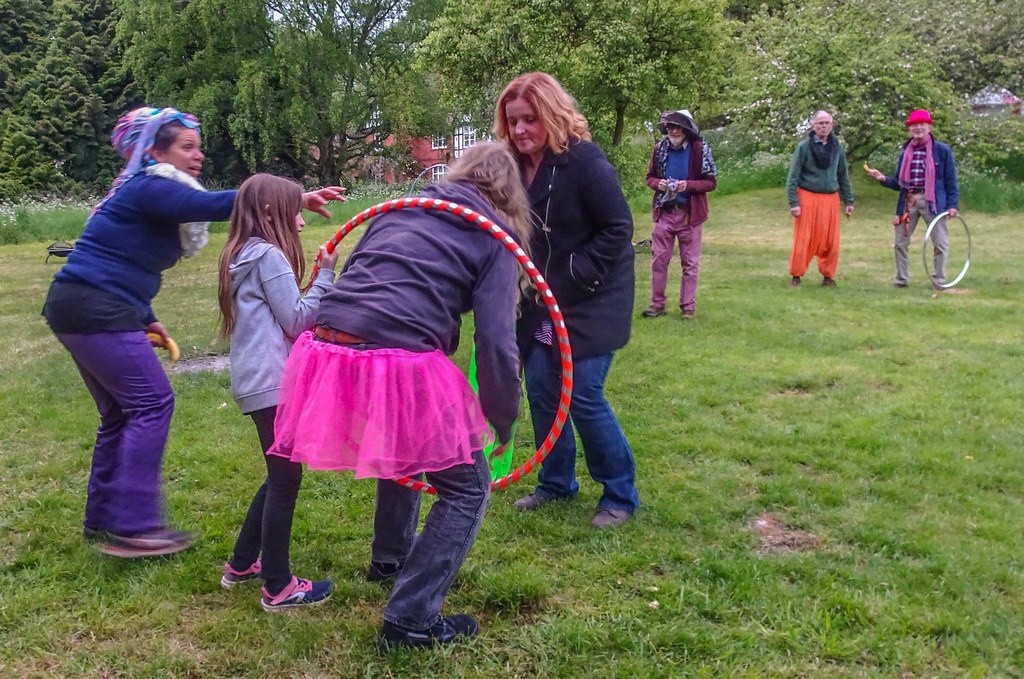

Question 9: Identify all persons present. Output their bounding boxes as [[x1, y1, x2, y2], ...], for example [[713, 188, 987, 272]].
[[268, 142, 535, 658], [866, 108, 960, 291], [493, 71, 639, 531], [39, 106, 349, 558], [785, 109, 855, 287], [215, 172, 338, 611], [641, 109, 717, 322]]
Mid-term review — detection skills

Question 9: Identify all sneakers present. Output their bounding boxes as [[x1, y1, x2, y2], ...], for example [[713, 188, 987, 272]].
[[378, 614, 480, 654], [260, 576, 333, 612], [82, 526, 197, 559], [367, 561, 401, 586], [220, 559, 262, 590]]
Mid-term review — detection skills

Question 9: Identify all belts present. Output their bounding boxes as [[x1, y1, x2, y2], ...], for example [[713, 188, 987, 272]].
[[908, 188, 925, 193]]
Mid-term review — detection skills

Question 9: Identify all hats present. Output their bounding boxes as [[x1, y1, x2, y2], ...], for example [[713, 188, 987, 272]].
[[656, 109, 699, 137], [903, 109, 933, 127]]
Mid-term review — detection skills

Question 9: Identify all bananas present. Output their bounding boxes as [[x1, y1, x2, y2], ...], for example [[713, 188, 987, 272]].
[[863, 161, 873, 172], [146, 332, 180, 363]]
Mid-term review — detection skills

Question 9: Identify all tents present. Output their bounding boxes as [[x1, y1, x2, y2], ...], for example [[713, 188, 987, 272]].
[[967, 83, 1024, 118]]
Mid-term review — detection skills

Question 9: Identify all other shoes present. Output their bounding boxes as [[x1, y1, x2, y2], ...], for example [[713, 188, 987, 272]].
[[681, 311, 694, 320], [823, 278, 836, 289], [791, 276, 800, 284], [642, 310, 664, 318], [512, 495, 555, 512], [932, 281, 943, 290], [591, 508, 629, 529], [894, 283, 909, 288]]
